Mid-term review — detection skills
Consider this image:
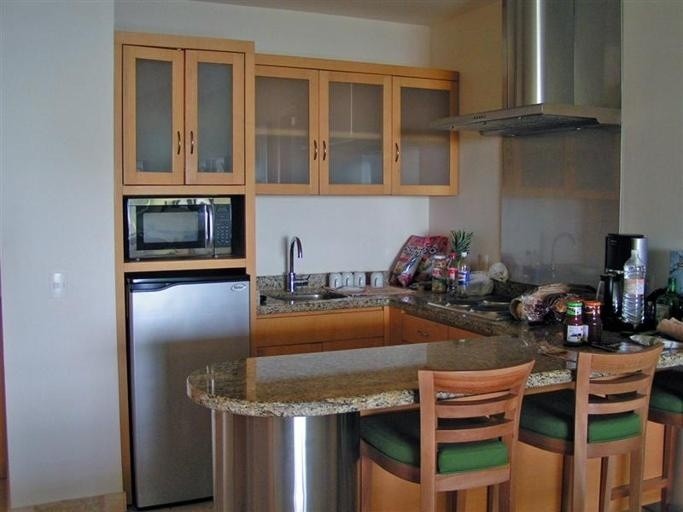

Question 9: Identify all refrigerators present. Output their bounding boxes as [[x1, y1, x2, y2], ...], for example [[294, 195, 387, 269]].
[[128, 277, 251, 507]]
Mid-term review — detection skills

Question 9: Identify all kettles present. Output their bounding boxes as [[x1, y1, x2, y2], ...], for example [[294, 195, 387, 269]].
[[594, 271, 620, 323]]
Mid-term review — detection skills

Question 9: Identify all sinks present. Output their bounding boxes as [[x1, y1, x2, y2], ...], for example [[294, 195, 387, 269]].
[[265, 293, 347, 300]]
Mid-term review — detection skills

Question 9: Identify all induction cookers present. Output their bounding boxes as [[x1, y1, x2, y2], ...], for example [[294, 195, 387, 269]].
[[426, 293, 523, 321]]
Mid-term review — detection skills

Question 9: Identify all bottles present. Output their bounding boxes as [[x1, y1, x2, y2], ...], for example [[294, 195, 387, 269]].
[[622, 250, 646, 327], [431, 250, 470, 293], [654, 276, 679, 326], [562, 296, 604, 349]]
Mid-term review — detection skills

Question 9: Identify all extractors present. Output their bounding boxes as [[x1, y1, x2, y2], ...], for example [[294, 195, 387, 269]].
[[427, 0, 622, 137]]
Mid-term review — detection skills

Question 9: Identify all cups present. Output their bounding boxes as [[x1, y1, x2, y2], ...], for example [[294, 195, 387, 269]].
[[353, 271, 365, 288], [341, 272, 353, 288], [478, 251, 492, 272], [329, 272, 341, 289], [369, 271, 384, 289]]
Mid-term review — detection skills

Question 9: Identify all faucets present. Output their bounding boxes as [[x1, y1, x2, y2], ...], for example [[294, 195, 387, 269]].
[[286, 235, 302, 291]]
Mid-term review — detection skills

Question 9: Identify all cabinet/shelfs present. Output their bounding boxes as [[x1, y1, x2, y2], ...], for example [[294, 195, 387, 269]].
[[257, 305, 488, 357]]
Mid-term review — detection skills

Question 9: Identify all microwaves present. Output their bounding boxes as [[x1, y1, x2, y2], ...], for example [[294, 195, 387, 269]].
[[127, 199, 231, 260]]
